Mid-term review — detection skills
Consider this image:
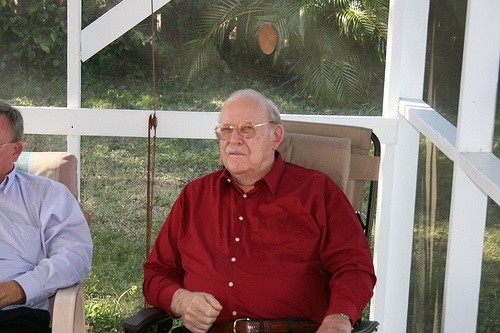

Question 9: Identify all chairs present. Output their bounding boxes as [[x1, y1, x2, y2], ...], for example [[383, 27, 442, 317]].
[[123, 119, 379, 332], [13, 152, 86, 333]]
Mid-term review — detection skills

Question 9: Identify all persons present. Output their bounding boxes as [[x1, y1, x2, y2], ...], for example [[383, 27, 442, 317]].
[[1, 99, 94, 333], [141, 88, 378, 333]]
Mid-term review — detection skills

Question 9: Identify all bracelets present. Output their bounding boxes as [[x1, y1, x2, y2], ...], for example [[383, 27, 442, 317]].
[[340, 312, 350, 319]]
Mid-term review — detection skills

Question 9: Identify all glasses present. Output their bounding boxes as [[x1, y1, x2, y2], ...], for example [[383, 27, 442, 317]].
[[215, 121, 274, 140]]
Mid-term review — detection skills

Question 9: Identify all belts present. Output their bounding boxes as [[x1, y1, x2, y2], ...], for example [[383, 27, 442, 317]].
[[205, 317, 318, 333]]
